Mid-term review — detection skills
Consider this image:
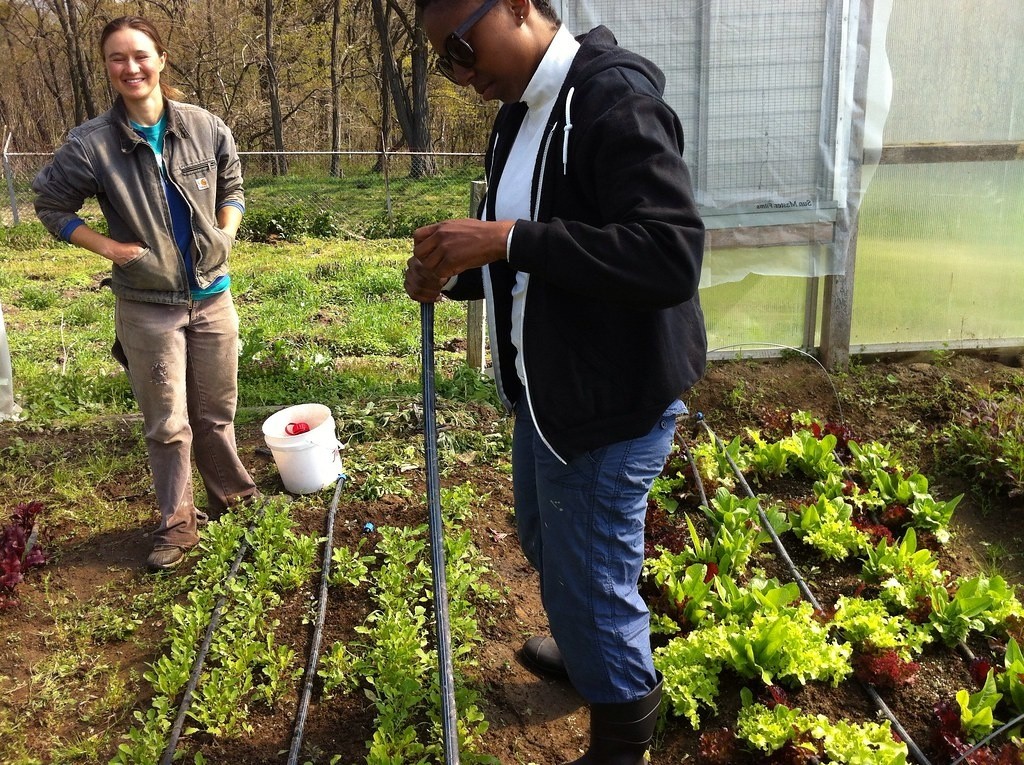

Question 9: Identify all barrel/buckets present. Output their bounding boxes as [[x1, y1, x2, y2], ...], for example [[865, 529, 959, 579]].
[[262, 404, 342, 494]]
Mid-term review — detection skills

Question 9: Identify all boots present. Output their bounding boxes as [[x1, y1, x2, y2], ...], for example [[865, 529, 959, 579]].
[[521, 636, 568, 680], [561, 668, 664, 765]]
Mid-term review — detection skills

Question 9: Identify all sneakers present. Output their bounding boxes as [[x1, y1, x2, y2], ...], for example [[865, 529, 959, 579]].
[[145, 537, 201, 570], [212, 494, 293, 517]]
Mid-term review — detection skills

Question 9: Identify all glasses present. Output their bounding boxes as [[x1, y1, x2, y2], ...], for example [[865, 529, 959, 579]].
[[435, 1, 498, 85]]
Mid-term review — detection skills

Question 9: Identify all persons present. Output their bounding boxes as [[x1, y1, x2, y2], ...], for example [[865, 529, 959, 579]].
[[404, 0, 708, 765], [33, 17, 293, 568]]
